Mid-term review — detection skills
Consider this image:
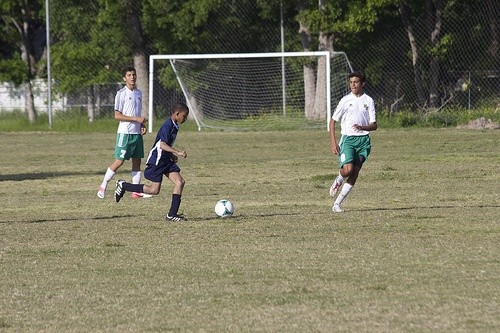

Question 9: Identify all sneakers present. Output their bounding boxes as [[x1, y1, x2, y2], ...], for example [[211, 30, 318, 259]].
[[167, 213, 188, 222], [97, 186, 106, 198], [132, 191, 152, 199], [115, 179, 126, 203], [332, 205, 345, 212], [330, 179, 343, 198]]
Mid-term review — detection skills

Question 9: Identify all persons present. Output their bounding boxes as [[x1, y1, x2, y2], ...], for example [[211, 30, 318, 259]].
[[329, 72, 377, 214], [96, 67, 157, 197], [116, 103, 189, 222]]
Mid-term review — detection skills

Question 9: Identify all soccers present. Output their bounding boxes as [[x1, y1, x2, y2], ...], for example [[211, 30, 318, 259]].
[[214, 199, 234, 219]]
